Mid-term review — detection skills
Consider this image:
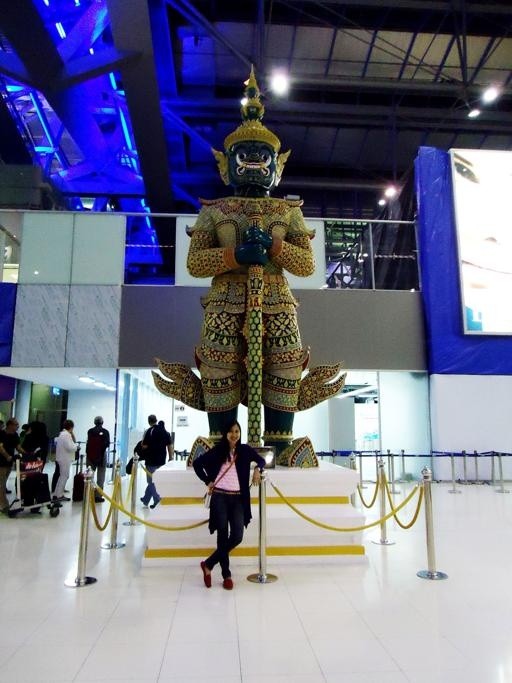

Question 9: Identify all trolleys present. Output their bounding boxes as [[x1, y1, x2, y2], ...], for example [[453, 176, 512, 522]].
[[7, 453, 63, 518]]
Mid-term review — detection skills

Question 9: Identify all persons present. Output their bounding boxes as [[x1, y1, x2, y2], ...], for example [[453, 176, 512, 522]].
[[0, 417, 49, 513], [158, 421, 165, 428], [53, 419, 81, 501], [193, 420, 266, 590], [86, 416, 110, 502], [52, 432, 76, 493], [186, 68, 317, 466], [141, 415, 173, 508]]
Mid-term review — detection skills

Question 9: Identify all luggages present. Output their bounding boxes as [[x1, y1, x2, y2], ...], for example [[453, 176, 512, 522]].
[[72, 449, 85, 502], [16, 471, 52, 506]]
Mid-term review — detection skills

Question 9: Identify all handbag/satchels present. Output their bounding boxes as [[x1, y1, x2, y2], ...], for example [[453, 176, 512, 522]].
[[204, 492, 212, 508]]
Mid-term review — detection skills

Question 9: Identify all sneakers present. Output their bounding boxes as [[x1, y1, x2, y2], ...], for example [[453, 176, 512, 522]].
[[223, 578, 233, 590], [51, 495, 71, 503], [95, 497, 105, 503], [201, 561, 212, 588], [150, 495, 161, 509], [140, 497, 149, 506]]
